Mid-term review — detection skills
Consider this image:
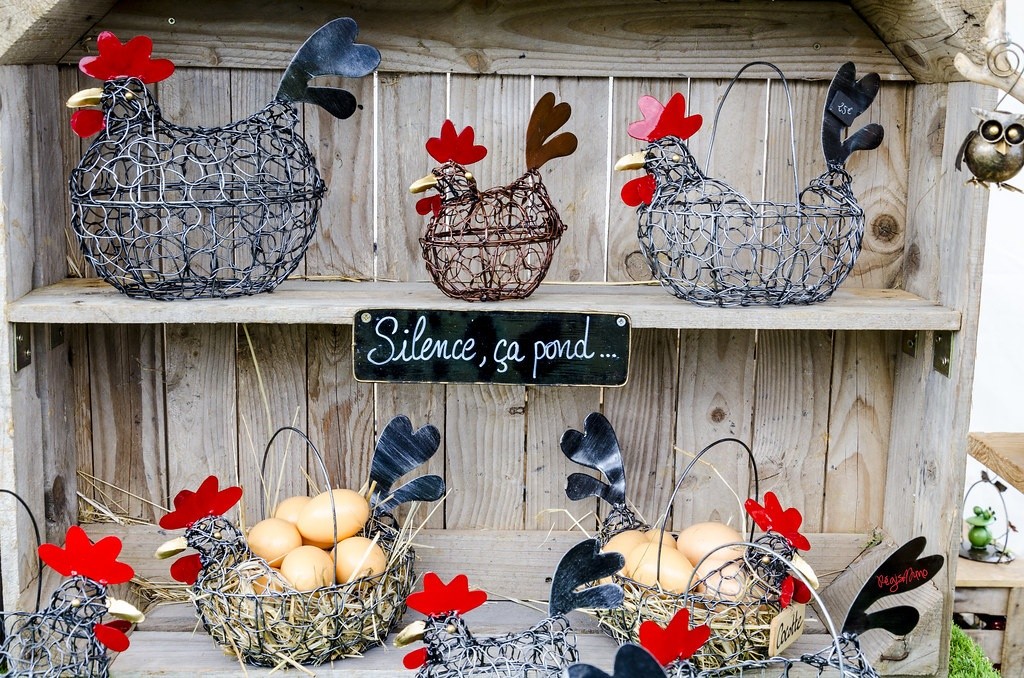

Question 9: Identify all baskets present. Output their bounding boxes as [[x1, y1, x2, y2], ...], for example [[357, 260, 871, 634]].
[[0, 488, 134, 678], [639, 542, 880, 678], [614, 61, 884, 309], [156, 412, 448, 671], [549, 411, 819, 678]]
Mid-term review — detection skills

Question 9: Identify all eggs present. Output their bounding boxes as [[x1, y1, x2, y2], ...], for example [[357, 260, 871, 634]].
[[600, 522, 773, 613], [247, 488, 386, 603]]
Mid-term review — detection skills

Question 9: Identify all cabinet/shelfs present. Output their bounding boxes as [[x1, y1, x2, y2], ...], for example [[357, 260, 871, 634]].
[[0, 0, 1005, 678]]
